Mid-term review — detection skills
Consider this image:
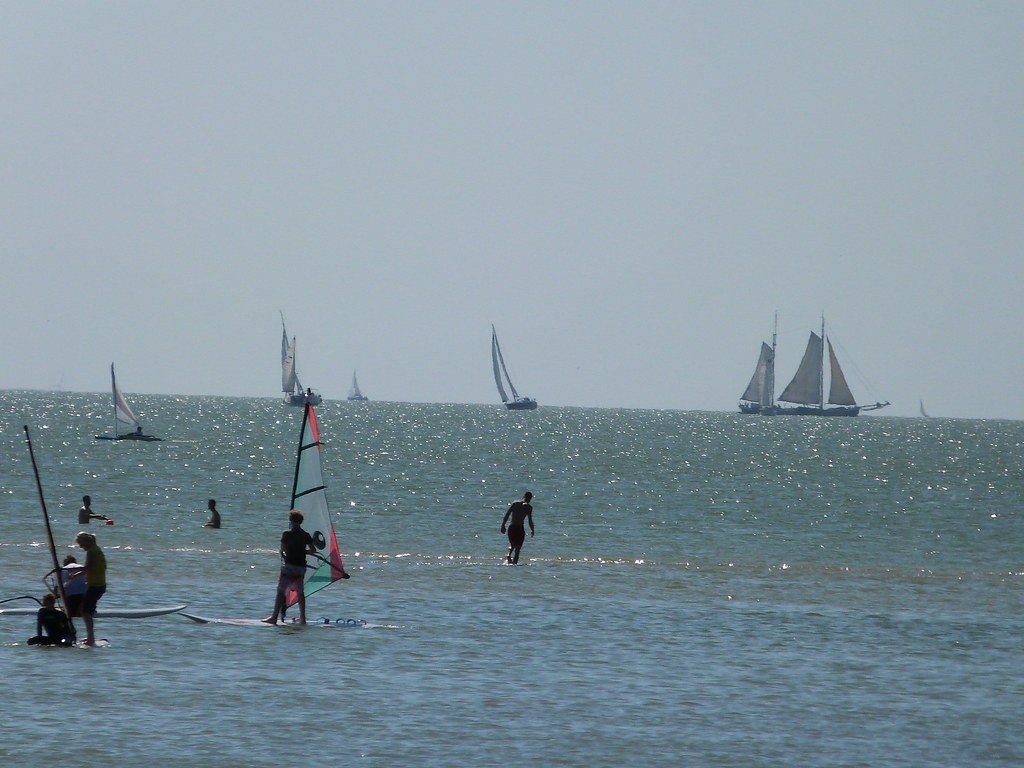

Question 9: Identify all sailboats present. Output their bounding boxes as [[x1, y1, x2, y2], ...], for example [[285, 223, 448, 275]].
[[490, 322, 539, 410], [278, 310, 323, 408], [92, 361, 162, 443], [346, 369, 368, 401], [738, 307, 891, 418]]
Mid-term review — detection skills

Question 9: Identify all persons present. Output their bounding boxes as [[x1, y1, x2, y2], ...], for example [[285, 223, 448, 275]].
[[203, 499, 220, 529], [261, 511, 316, 625], [61, 555, 89, 616], [132, 426, 143, 435], [79, 495, 110, 524], [26, 593, 74, 646], [500, 492, 534, 564], [68, 532, 107, 646], [304, 388, 314, 396]]
